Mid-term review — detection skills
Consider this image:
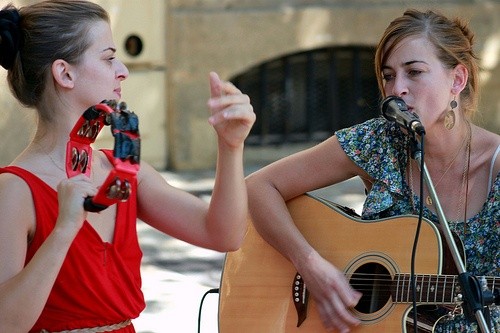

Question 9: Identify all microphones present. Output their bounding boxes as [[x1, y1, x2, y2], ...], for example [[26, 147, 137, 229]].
[[380, 95, 426, 134]]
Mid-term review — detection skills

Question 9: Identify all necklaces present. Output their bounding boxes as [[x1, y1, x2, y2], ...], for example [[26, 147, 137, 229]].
[[36, 134, 70, 174], [407, 119, 474, 244]]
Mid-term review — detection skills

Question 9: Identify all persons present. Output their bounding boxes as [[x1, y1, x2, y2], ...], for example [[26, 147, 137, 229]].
[[245, 9, 500, 333], [0, 1, 255, 333]]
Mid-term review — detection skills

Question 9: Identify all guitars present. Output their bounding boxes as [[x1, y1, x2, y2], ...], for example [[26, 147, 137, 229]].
[[218, 194, 500, 333]]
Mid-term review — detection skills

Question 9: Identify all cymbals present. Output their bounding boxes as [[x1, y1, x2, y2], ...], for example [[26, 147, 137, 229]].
[[65, 99, 141, 212]]
